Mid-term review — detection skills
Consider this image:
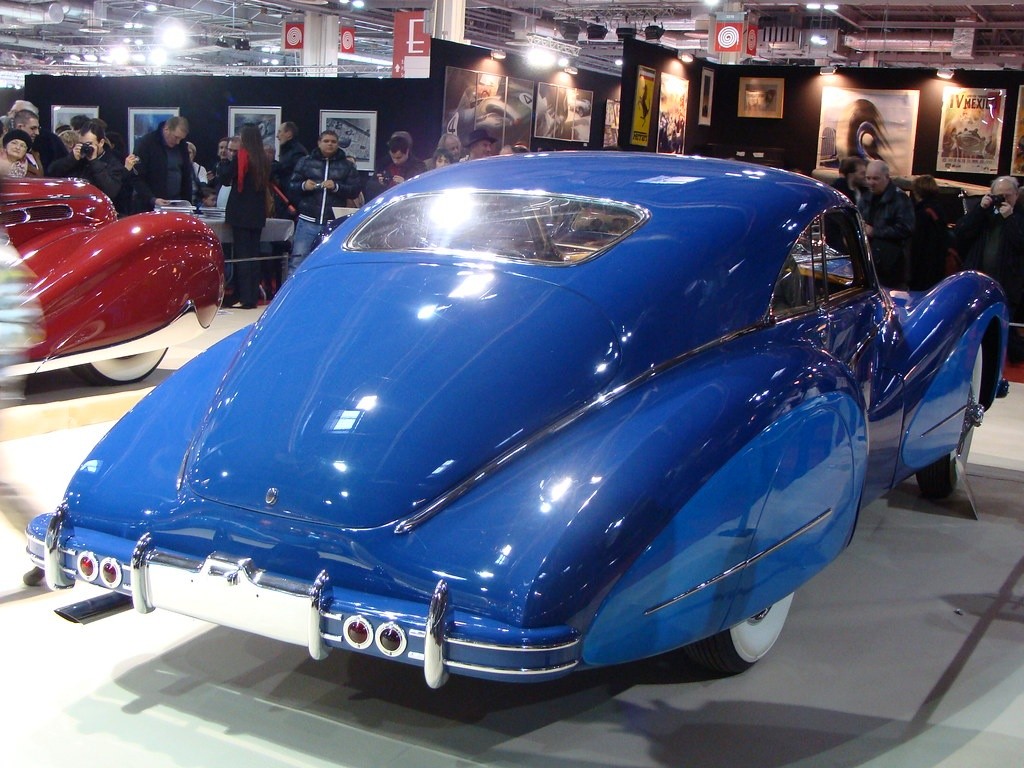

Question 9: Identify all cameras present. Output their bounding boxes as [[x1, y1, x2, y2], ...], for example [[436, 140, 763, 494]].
[[316, 184, 321, 188], [382, 175, 393, 183], [80, 142, 94, 157], [135, 156, 141, 164], [990, 194, 1005, 207]]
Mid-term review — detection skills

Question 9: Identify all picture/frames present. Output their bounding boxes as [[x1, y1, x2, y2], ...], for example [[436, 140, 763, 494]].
[[737, 77, 784, 120], [51, 104, 100, 134], [656, 72, 689, 157], [319, 109, 379, 172], [227, 105, 283, 161], [127, 106, 180, 156], [698, 65, 715, 128]]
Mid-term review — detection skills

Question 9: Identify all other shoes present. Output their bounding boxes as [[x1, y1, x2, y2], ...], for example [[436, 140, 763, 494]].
[[1007, 338, 1024, 364], [249, 304, 257, 308], [231, 300, 251, 309]]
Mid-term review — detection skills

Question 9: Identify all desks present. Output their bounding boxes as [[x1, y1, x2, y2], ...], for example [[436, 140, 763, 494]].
[[196, 213, 296, 291]]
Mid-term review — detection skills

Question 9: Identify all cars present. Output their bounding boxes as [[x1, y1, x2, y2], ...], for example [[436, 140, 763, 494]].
[[19, 152, 1011, 690], [568, 92, 591, 116], [0, 174, 225, 395]]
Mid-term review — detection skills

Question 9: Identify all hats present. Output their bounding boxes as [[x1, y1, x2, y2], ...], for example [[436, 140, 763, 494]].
[[462, 128, 498, 147], [3, 128, 33, 152], [388, 130, 413, 146]]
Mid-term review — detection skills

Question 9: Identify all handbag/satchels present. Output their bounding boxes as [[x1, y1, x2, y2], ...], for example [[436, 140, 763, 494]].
[[266, 188, 275, 217]]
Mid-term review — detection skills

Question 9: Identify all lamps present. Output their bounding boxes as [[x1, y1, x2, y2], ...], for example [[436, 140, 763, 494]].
[[586, 19, 609, 41], [234, 30, 252, 50], [214, 35, 230, 48], [247, 18, 254, 30], [559, 18, 581, 42], [615, 23, 638, 41], [565, 66, 578, 76], [681, 52, 694, 63], [490, 49, 507, 59], [935, 68, 955, 79], [819, 67, 838, 76], [644, 24, 666, 40]]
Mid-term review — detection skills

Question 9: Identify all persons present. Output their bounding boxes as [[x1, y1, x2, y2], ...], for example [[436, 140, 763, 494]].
[[217, 122, 530, 218], [288, 130, 361, 283], [216, 123, 270, 310], [957, 177, 1024, 368], [825, 157, 957, 292], [1, 100, 209, 220]]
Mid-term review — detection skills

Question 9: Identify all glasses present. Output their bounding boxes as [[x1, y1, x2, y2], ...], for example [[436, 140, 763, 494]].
[[30, 126, 41, 130], [390, 145, 407, 153], [172, 134, 179, 140]]
